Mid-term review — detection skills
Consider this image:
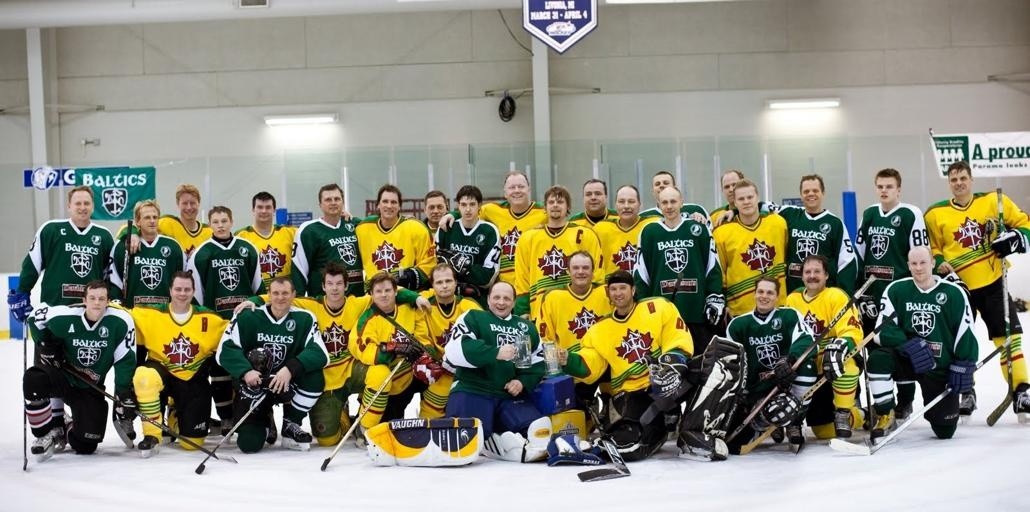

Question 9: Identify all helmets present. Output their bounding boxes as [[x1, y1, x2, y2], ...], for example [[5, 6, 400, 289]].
[[246, 346, 274, 377]]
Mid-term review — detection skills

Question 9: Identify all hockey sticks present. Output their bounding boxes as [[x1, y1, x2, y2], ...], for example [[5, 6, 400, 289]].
[[828, 341, 1008, 454], [196, 391, 269, 474], [578, 404, 631, 483], [727, 310, 899, 455], [59, 364, 238, 464], [319, 356, 406, 470], [112, 402, 135, 449], [22, 319, 28, 470], [986, 187, 1013, 427], [862, 346, 874, 447]]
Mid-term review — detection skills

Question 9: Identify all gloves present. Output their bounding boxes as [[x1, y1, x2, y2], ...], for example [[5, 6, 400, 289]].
[[774, 354, 796, 387], [7, 291, 33, 321], [821, 338, 849, 381], [649, 351, 688, 401], [392, 267, 410, 288], [858, 295, 879, 320], [990, 231, 1022, 259], [437, 247, 470, 281], [902, 339, 935, 373], [380, 341, 425, 363], [704, 293, 726, 326], [940, 272, 972, 298], [947, 359, 978, 393]]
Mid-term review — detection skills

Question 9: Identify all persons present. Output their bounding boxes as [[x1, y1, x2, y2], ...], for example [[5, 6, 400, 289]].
[[107, 196, 186, 451], [115, 181, 215, 266], [24, 280, 138, 462], [232, 260, 433, 448], [215, 277, 332, 454], [233, 191, 301, 297], [289, 184, 365, 299], [345, 161, 1030, 481], [106, 270, 231, 458], [8, 184, 115, 452], [187, 204, 268, 441]]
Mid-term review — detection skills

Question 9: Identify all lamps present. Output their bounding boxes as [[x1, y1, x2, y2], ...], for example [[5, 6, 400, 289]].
[[264, 113, 339, 126], [766, 97, 844, 111]]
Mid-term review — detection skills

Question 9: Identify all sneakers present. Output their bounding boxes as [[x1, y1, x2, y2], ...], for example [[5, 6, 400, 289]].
[[30, 396, 313, 455], [769, 389, 1030, 447]]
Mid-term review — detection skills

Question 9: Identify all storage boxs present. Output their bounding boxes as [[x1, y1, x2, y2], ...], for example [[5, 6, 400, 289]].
[[551, 409, 588, 442], [533, 373, 576, 414]]
